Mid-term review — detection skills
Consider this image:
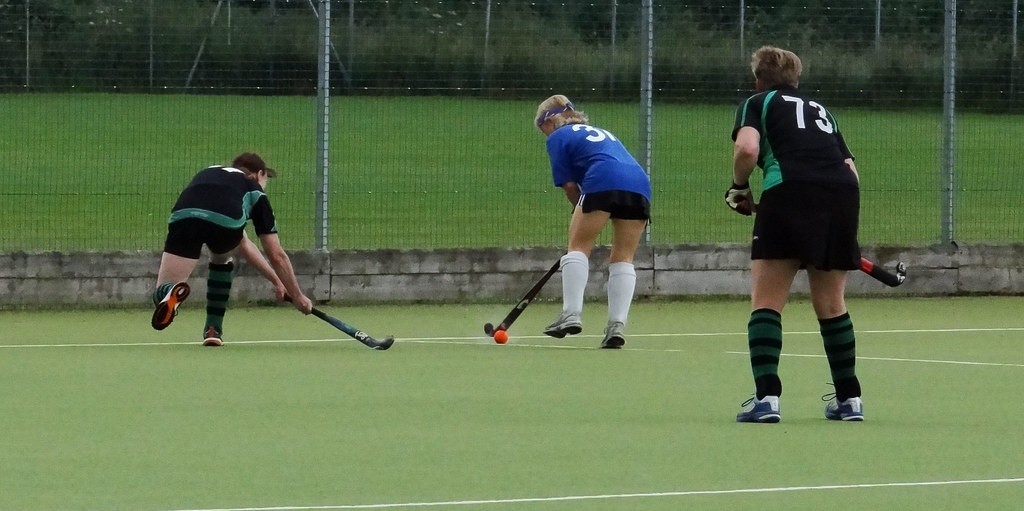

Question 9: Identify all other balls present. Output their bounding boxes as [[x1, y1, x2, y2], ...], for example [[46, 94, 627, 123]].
[[493, 330, 509, 344]]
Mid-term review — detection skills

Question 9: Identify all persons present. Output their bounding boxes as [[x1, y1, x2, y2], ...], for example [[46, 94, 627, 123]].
[[150, 152, 313, 347], [724, 46, 865, 424], [535, 94, 651, 349]]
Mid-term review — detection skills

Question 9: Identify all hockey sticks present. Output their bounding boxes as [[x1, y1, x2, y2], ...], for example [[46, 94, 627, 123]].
[[732, 194, 907, 287], [483, 252, 567, 337], [282, 291, 395, 351]]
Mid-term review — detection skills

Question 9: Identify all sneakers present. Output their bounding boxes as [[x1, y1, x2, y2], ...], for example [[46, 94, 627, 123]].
[[203, 326, 224, 347], [544, 312, 582, 338], [822, 383, 863, 421], [736, 393, 781, 422], [601, 321, 626, 348], [152, 281, 190, 330]]
